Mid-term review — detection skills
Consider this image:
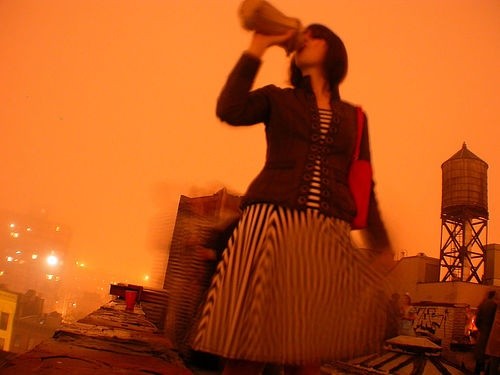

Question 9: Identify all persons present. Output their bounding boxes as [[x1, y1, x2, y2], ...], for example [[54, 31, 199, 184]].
[[188, 20, 410, 374]]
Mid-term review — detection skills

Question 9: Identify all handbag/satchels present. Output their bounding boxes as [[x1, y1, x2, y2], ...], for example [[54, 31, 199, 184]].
[[349, 106, 374, 229]]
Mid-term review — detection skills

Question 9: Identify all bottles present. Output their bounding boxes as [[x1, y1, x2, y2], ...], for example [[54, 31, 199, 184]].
[[238, 0, 305, 57]]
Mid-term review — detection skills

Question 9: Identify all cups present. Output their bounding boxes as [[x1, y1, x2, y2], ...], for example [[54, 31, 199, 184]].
[[125, 288, 138, 310]]
